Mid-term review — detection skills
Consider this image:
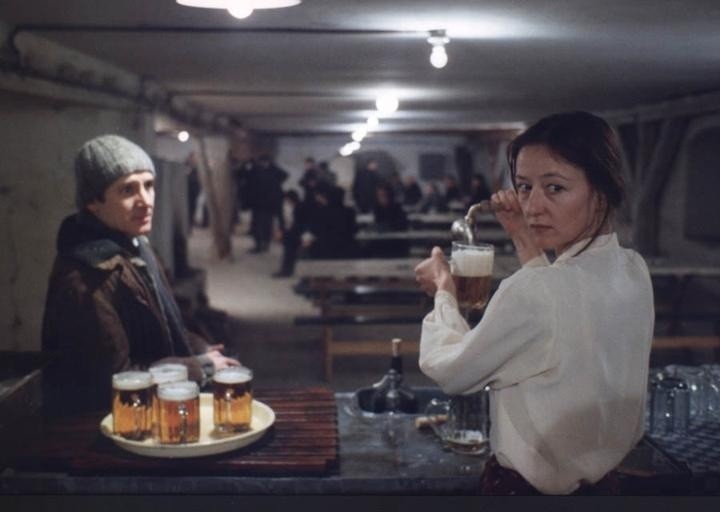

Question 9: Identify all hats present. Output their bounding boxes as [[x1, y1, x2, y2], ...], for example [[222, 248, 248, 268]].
[[75, 134, 156, 206]]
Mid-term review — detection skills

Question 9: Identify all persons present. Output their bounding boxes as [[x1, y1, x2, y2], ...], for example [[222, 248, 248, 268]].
[[415, 111, 655, 498], [185, 144, 494, 267], [42, 134, 241, 414]]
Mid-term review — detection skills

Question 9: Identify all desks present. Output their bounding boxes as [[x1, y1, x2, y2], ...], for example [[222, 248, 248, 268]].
[[282, 212, 719, 382]]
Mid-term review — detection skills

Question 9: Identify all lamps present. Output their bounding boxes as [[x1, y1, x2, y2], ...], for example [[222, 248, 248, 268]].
[[423, 31, 450, 69]]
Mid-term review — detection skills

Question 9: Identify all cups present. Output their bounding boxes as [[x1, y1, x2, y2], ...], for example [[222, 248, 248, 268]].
[[111, 363, 201, 446], [212, 367, 255, 434], [426, 391, 489, 457], [645, 360, 720, 441], [448, 239, 493, 313]]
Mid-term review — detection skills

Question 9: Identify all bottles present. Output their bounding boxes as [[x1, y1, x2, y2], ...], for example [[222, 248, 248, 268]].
[[369, 337, 417, 413]]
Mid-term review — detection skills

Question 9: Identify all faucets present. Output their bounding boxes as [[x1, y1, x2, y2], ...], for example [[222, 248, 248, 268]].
[[450, 200, 507, 245]]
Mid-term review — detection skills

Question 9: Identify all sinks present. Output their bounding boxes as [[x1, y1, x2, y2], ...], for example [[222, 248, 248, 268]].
[[353, 386, 490, 424]]
